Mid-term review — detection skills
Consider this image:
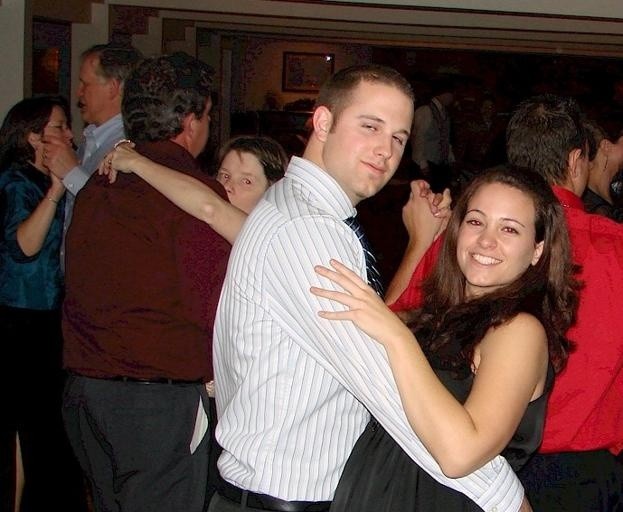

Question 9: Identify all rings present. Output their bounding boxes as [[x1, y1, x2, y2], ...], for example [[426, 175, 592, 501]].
[[103, 153, 108, 159], [109, 160, 112, 164]]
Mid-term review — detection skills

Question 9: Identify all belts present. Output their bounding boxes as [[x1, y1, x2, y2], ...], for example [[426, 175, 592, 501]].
[[218, 482, 332, 512], [69, 373, 203, 385]]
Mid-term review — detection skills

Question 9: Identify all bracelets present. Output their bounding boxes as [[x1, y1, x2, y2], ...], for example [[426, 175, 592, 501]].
[[114, 138, 135, 149], [44, 196, 58, 205]]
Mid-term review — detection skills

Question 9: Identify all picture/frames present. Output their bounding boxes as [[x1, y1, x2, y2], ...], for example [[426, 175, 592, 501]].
[[282, 51, 335, 94]]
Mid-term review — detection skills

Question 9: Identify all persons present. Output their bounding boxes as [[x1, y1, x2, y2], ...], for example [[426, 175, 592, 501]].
[[310, 164, 585, 512], [386, 96, 623, 512], [41, 45, 147, 277], [61, 52, 232, 512], [98, 137, 288, 246], [208, 64, 535, 512], [410, 85, 455, 194], [0, 95, 82, 512], [581, 103, 623, 223]]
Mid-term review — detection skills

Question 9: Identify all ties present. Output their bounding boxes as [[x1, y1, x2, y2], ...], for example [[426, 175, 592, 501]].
[[342, 216, 384, 301]]
